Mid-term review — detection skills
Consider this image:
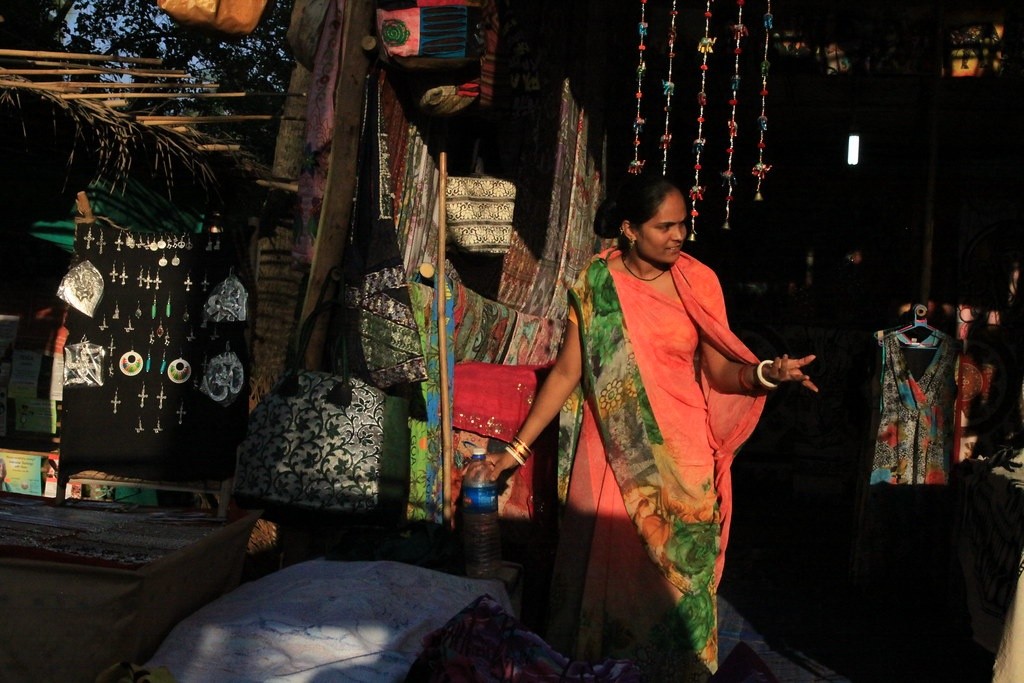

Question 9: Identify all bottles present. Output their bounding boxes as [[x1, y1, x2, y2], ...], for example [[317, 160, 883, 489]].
[[463, 454, 500, 578]]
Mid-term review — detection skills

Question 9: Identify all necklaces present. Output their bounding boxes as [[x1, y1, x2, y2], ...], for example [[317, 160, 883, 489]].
[[622, 251, 666, 281]]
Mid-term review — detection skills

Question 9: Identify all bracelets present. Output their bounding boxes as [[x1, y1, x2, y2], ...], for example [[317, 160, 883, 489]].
[[756, 359, 778, 390], [505, 436, 532, 467]]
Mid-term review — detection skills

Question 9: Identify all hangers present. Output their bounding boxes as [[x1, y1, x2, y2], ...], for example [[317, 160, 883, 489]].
[[875, 305, 945, 350]]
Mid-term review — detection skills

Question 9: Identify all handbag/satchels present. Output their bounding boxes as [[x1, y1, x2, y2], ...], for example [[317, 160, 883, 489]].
[[229, 267, 411, 518]]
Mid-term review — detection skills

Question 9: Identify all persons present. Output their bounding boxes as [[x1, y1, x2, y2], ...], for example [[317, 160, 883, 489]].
[[460, 178, 820, 683]]
